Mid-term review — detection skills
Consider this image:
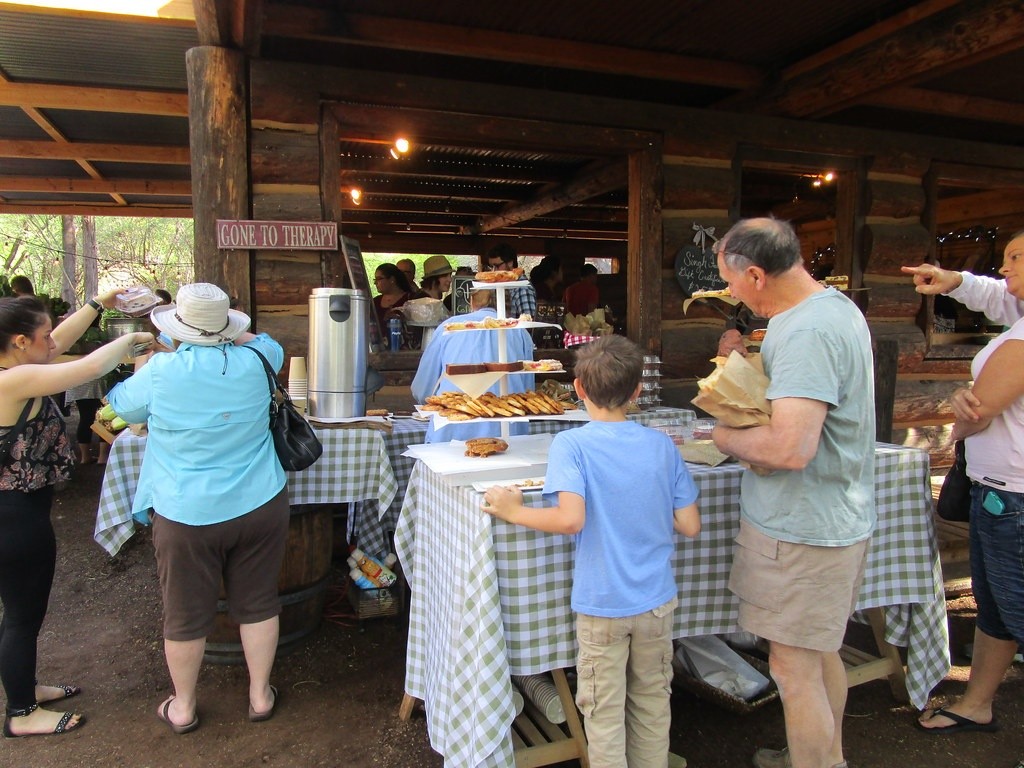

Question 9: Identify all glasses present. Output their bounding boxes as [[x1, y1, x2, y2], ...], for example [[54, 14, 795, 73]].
[[487, 260, 510, 271], [711, 239, 750, 268], [373, 277, 391, 283]]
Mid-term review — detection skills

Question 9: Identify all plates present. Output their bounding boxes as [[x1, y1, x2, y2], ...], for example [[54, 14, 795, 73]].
[[473, 476, 545, 491]]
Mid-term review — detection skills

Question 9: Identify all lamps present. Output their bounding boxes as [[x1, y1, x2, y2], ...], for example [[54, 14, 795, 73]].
[[389, 136, 412, 159], [352, 184, 368, 206]]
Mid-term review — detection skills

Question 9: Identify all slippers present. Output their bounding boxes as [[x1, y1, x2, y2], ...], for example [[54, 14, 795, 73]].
[[80, 456, 98, 465], [248, 686, 277, 722], [913, 706, 999, 735], [96, 462, 107, 466], [158, 697, 199, 734]]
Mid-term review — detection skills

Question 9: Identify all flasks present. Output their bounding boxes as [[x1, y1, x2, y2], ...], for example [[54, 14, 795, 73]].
[[389, 318, 402, 352]]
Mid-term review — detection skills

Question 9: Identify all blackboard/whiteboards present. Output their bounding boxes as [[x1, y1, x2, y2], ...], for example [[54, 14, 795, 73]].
[[674, 243, 729, 304]]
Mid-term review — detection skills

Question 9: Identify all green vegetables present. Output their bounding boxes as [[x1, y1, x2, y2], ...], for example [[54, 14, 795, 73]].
[[0, 274, 124, 354]]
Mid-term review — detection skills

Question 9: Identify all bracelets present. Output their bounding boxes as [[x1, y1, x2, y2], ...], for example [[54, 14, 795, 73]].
[[87, 299, 104, 313]]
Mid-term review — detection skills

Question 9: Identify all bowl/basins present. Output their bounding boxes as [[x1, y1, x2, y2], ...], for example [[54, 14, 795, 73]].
[[117, 287, 151, 310]]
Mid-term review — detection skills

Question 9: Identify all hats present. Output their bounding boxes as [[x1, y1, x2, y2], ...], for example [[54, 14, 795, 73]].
[[150, 282, 251, 347], [421, 256, 457, 279]]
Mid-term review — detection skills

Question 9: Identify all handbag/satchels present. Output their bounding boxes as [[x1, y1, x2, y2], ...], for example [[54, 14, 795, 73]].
[[937, 440, 972, 522], [243, 346, 323, 471]]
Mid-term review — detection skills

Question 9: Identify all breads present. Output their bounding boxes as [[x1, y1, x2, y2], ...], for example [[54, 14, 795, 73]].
[[366, 409, 389, 415], [749, 328, 768, 341], [446, 359, 564, 374], [717, 328, 746, 359], [118, 288, 152, 311], [421, 391, 577, 422], [476, 267, 524, 283], [445, 313, 533, 329], [465, 436, 508, 458]]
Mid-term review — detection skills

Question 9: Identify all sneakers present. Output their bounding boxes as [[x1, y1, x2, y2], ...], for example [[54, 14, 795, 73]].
[[751, 747, 848, 768]]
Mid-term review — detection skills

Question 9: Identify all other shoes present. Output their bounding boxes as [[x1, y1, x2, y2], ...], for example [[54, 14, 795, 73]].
[[963, 643, 1024, 664]]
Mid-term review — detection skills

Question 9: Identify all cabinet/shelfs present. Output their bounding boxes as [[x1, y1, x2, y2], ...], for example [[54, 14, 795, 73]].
[[442, 282, 567, 472]]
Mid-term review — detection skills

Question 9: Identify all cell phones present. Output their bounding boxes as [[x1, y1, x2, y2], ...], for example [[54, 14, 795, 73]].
[[984, 492, 1006, 514]]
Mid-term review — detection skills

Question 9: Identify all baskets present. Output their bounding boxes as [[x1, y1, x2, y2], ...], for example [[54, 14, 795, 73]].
[[672, 647, 781, 716]]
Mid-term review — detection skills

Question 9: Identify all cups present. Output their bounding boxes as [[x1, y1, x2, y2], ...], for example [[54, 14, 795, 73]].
[[512, 674, 566, 723], [288, 357, 307, 417]]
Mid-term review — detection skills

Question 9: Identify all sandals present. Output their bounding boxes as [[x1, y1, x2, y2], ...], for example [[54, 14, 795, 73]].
[[3, 701, 85, 738], [35, 677, 80, 706]]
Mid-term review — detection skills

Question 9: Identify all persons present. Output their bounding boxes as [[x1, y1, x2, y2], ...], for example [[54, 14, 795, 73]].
[[915, 232, 1024, 735], [108, 282, 290, 732], [373, 238, 601, 351], [712, 213, 876, 768], [0, 275, 172, 740], [900, 263, 1024, 328], [410, 288, 536, 444], [479, 335, 706, 768]]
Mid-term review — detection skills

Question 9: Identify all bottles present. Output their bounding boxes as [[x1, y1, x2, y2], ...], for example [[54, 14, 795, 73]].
[[347, 548, 397, 611]]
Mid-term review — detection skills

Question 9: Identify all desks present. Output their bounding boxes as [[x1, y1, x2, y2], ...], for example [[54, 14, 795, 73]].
[[93, 403, 951, 768]]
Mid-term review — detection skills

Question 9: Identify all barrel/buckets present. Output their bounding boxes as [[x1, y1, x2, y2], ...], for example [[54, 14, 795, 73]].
[[205, 501, 332, 663], [306, 287, 371, 422]]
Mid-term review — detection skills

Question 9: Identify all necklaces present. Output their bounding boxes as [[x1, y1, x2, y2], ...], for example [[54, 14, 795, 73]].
[[387, 299, 391, 301]]
